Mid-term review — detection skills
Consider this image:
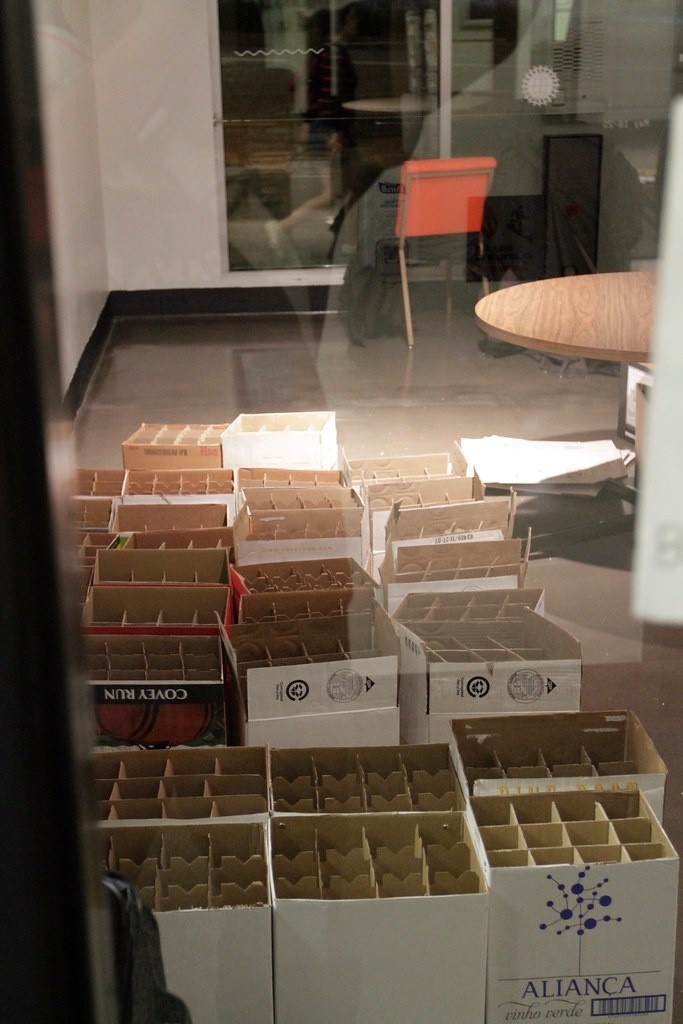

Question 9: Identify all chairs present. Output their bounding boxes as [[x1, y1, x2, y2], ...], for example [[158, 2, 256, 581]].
[[364, 154, 507, 352]]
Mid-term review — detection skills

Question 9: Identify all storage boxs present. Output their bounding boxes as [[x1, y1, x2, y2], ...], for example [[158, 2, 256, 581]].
[[55, 402, 683, 1024]]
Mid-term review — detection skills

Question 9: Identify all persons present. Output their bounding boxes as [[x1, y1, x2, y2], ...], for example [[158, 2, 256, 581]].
[[468, 92, 649, 360], [264, 7, 364, 256], [331, 129, 454, 348]]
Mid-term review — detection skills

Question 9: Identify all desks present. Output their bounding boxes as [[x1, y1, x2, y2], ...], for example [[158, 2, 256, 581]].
[[474, 269, 657, 364]]
